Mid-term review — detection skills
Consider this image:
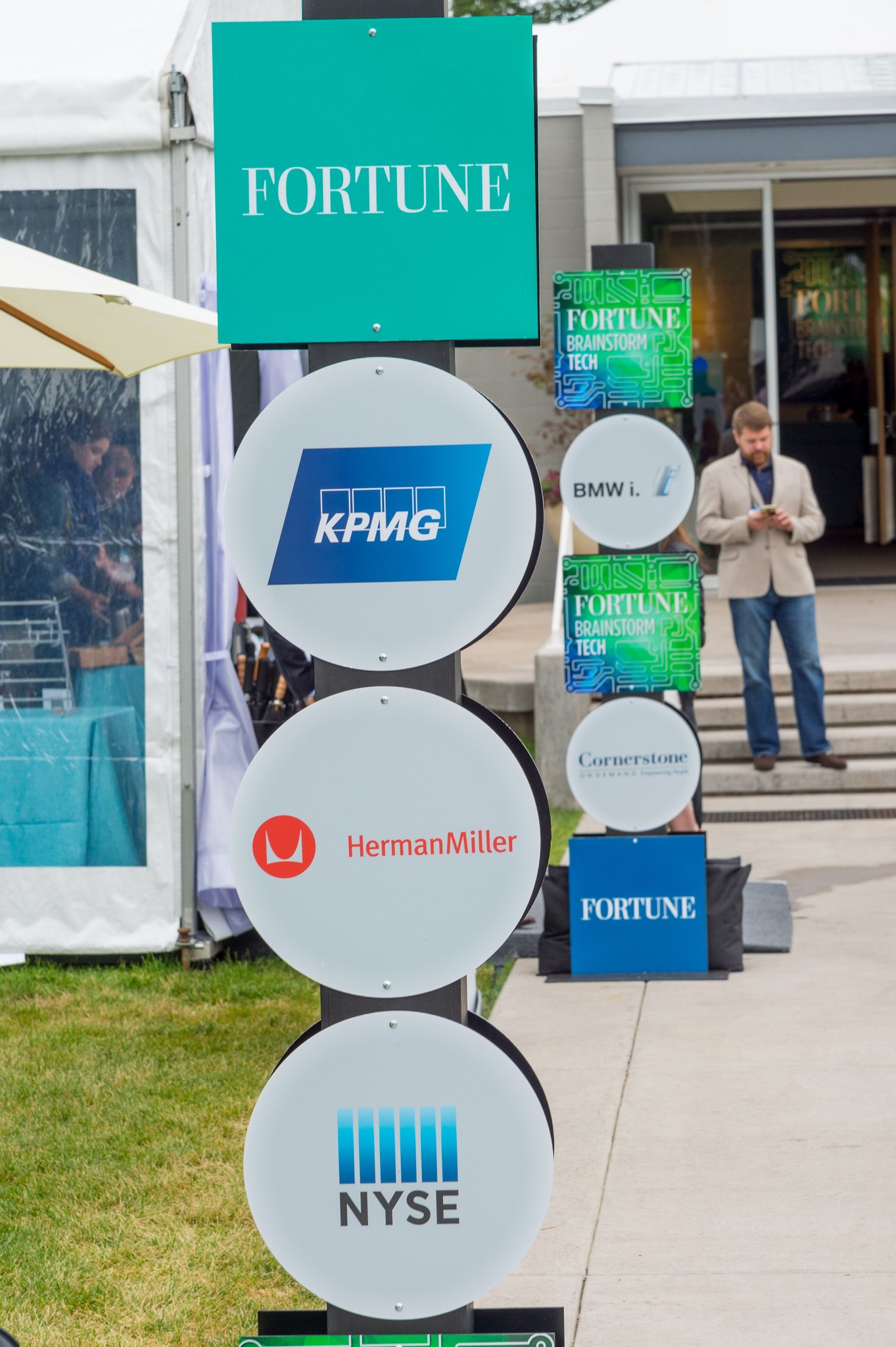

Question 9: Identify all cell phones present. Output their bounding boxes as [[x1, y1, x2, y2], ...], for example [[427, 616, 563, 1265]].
[[759, 503, 776, 517]]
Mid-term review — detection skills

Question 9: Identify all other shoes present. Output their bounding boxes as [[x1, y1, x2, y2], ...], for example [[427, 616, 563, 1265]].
[[804, 752, 846, 769], [753, 755, 776, 769]]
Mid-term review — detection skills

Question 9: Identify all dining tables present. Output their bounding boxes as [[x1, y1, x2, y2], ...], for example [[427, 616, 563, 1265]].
[[0, 705, 147, 866], [74, 662, 146, 717]]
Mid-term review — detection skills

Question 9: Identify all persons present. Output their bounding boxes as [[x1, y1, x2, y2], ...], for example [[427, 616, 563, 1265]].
[[261, 616, 316, 705], [692, 400, 849, 773], [82, 438, 142, 632], [666, 353, 728, 485], [23, 411, 142, 686]]
